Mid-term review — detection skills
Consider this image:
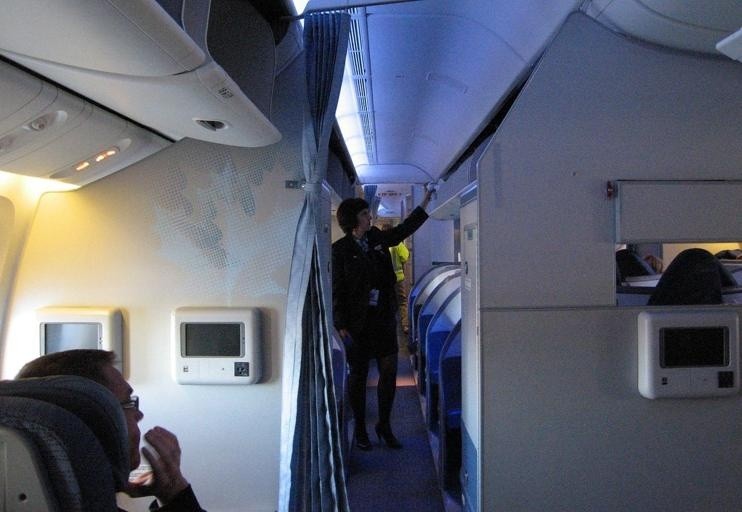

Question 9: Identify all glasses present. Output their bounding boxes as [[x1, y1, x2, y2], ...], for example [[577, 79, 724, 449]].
[[123, 395, 139, 413]]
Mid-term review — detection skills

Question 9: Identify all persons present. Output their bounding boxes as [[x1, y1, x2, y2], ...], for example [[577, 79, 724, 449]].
[[642, 254, 666, 274], [331, 182, 433, 450], [382, 224, 410, 334], [15, 349, 206, 512]]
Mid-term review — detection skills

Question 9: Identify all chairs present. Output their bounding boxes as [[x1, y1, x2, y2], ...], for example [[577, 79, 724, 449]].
[[0, 375, 132, 511], [408, 261, 463, 502]]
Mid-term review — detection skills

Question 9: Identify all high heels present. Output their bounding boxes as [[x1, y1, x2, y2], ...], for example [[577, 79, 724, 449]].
[[375, 422, 403, 449], [356, 429, 371, 450]]
